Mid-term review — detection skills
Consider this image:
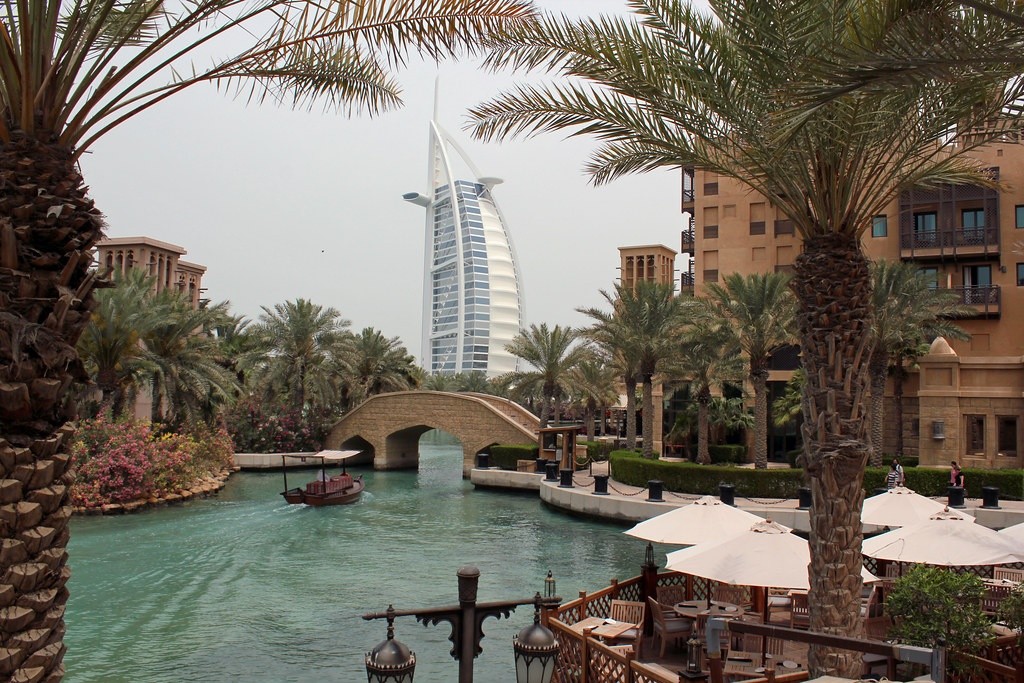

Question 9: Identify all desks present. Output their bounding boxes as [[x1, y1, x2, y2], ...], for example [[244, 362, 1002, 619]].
[[766, 596, 791, 606], [674, 600, 745, 637], [872, 575, 899, 587], [787, 590, 809, 598], [991, 620, 1022, 636], [977, 577, 1022, 594], [570, 617, 637, 642], [720, 650, 801, 678]]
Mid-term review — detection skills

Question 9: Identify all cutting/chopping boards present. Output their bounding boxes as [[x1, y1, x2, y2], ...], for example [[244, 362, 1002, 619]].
[[591, 621, 636, 639]]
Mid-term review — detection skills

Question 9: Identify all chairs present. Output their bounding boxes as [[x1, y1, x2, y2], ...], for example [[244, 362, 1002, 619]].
[[589, 567, 1024, 683]]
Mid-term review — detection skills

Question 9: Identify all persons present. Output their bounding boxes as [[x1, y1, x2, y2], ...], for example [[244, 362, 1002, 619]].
[[885, 459, 904, 490], [950, 461, 964, 488]]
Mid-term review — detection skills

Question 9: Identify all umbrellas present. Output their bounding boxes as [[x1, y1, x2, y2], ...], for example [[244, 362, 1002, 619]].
[[624, 494, 794, 611], [861, 485, 976, 583], [860, 509, 1024, 577], [663, 519, 880, 668]]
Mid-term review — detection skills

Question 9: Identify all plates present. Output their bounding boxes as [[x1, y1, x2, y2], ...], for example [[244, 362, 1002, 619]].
[[783, 661, 797, 669], [725, 607, 736, 612]]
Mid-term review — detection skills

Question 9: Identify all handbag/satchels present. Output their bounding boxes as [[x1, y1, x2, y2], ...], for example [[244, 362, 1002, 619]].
[[898, 482, 903, 487], [898, 465, 905, 482]]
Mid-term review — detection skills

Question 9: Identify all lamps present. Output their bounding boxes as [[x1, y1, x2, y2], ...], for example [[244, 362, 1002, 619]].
[[365, 617, 417, 683], [512, 609, 561, 683]]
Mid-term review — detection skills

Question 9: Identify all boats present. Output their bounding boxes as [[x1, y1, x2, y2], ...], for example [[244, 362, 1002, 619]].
[[279, 446, 367, 507]]
